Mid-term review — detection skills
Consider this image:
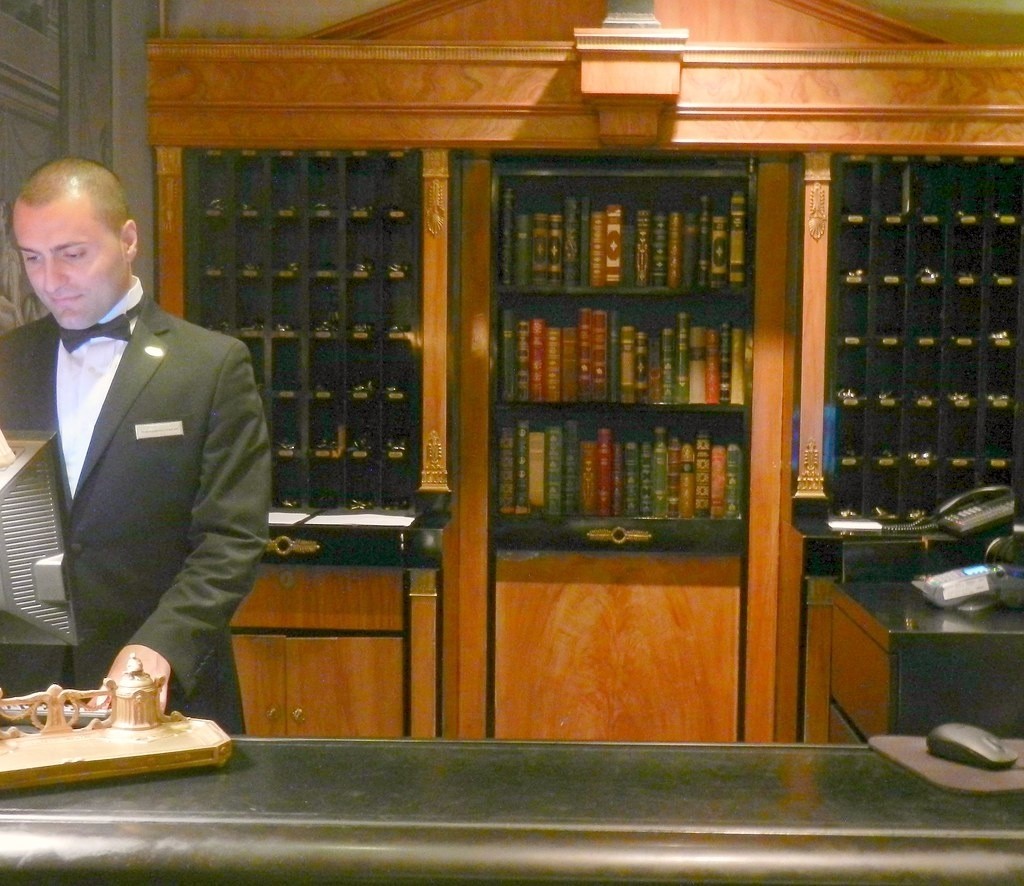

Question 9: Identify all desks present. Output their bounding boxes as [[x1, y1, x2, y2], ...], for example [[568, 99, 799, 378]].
[[1, 722, 1023, 886]]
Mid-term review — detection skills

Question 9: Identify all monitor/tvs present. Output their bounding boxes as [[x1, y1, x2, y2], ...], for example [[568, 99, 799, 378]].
[[0, 429, 79, 646]]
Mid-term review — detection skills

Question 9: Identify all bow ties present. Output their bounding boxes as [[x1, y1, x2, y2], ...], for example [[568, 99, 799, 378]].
[[59, 314, 133, 353]]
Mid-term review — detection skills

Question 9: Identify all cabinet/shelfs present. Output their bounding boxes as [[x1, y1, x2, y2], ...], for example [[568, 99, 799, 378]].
[[145, 0, 1024, 746], [830, 581, 1024, 746]]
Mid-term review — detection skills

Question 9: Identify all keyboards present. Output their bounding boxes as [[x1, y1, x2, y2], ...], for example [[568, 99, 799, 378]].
[[2, 705, 112, 728]]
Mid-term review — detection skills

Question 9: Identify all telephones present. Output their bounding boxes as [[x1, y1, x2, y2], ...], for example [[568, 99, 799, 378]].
[[934, 484, 1015, 537]]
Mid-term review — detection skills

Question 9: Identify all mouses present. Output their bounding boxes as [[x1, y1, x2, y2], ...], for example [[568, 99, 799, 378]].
[[925, 723, 1021, 768]]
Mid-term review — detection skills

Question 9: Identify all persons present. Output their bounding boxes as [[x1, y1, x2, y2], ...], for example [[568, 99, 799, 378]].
[[0, 159, 273, 736]]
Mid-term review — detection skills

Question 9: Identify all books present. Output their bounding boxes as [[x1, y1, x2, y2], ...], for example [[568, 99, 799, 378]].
[[499, 304, 747, 406], [500, 183, 748, 287], [498, 419, 740, 519]]
